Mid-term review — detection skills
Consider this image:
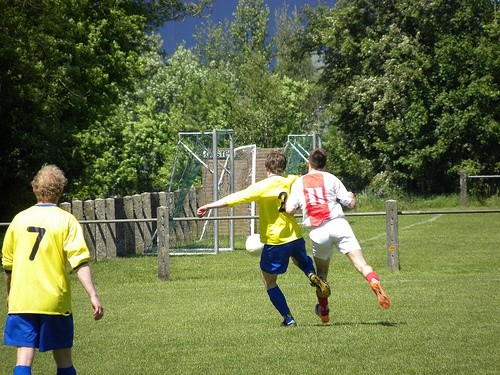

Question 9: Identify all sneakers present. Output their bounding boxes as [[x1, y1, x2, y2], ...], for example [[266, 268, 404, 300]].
[[308, 274, 331, 298], [280, 316, 298, 327], [315, 303, 330, 323], [370, 278, 390, 309]]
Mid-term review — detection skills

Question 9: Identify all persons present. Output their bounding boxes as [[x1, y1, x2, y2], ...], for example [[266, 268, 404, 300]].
[[286, 150, 390, 323], [1, 163, 104, 375], [197, 151, 330, 328]]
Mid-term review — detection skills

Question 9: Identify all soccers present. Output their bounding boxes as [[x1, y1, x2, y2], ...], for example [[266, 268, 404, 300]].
[[245, 234, 265, 257]]
